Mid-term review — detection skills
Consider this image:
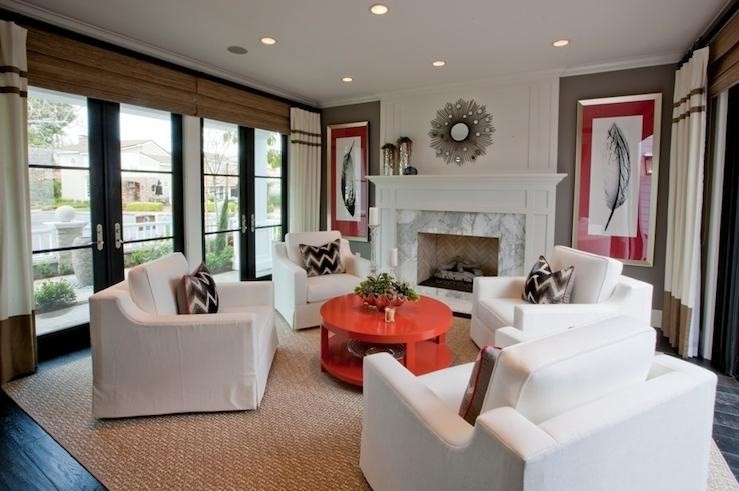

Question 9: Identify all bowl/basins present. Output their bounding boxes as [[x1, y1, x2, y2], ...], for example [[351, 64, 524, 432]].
[[347, 338, 405, 361]]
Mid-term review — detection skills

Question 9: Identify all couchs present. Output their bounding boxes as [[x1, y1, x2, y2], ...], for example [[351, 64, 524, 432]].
[[271, 227, 375, 333], [359, 329, 721, 491], [467, 240, 657, 357], [87, 251, 281, 421]]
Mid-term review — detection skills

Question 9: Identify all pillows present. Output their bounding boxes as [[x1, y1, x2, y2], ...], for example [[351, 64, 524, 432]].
[[173, 259, 221, 315], [455, 343, 505, 426], [519, 253, 576, 304], [298, 238, 345, 278]]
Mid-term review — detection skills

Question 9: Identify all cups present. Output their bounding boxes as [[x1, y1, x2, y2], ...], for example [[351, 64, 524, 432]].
[[384, 306, 396, 324]]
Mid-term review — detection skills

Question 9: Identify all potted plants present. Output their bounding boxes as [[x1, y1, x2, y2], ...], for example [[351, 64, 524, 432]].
[[353, 271, 422, 313]]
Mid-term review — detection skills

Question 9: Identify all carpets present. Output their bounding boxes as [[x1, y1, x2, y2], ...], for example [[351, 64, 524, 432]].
[[1, 312, 738, 489]]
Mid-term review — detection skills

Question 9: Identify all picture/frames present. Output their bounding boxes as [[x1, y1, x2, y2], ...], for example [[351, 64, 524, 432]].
[[324, 119, 373, 243], [569, 89, 665, 269]]
